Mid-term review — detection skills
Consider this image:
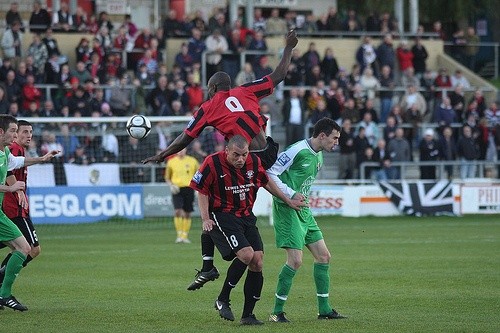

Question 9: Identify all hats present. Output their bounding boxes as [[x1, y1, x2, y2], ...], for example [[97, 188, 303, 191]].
[[102, 102, 109, 110], [424, 128, 434, 136]]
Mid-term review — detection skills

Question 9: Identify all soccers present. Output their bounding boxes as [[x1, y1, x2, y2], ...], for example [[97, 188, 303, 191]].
[[125, 114, 152, 140]]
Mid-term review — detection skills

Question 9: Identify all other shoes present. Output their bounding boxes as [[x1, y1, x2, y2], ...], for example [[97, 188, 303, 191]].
[[175, 238, 182, 242], [184, 239, 191, 243]]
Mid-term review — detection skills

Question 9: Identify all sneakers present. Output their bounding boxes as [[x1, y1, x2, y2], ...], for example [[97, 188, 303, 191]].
[[214, 296, 234, 322], [0, 266, 6, 288], [187, 265, 220, 291], [268, 312, 289, 323], [318, 309, 344, 319], [0, 294, 28, 312], [241, 314, 264, 324]]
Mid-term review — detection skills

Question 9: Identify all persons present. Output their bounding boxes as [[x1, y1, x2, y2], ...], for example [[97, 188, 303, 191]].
[[265, 118, 345, 322], [0, 115, 60, 312], [189, 134, 310, 324], [162, 6, 286, 161], [165, 147, 200, 243], [0, 0, 170, 181], [282, 5, 500, 180], [140, 28, 298, 291]]
